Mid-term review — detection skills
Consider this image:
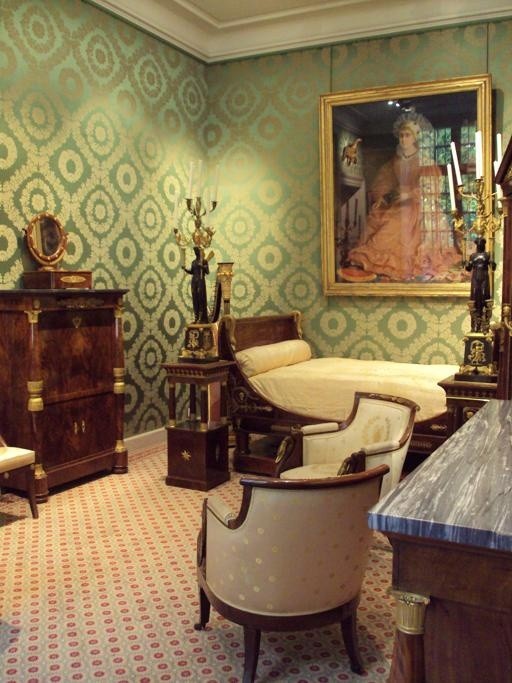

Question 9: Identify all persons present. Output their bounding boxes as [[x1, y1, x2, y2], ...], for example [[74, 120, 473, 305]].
[[344, 112, 462, 278]]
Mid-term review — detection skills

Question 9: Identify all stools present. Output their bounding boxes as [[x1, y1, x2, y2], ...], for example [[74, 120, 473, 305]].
[[0, 437, 40, 519]]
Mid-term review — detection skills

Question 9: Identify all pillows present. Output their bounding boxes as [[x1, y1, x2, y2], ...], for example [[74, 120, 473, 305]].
[[234, 339, 312, 378]]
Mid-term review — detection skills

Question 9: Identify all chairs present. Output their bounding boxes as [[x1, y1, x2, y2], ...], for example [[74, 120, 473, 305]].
[[197, 464, 391, 682], [275, 390, 421, 504]]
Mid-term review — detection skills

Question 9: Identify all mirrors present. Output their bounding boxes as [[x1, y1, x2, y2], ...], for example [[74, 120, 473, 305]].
[[20, 212, 69, 270]]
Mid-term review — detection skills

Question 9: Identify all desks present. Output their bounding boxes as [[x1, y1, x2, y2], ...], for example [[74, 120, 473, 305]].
[[366, 401, 512, 681]]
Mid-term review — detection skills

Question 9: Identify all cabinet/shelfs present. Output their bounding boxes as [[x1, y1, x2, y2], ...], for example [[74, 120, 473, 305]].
[[437, 375, 498, 437], [494, 135, 512, 400], [161, 360, 237, 492], [0, 288, 131, 504]]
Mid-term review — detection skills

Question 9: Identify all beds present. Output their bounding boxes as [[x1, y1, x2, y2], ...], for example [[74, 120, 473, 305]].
[[217, 310, 460, 478]]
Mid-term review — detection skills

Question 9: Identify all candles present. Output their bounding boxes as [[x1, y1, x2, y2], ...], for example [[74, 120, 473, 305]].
[[445, 131, 504, 211], [186, 159, 221, 202]]
